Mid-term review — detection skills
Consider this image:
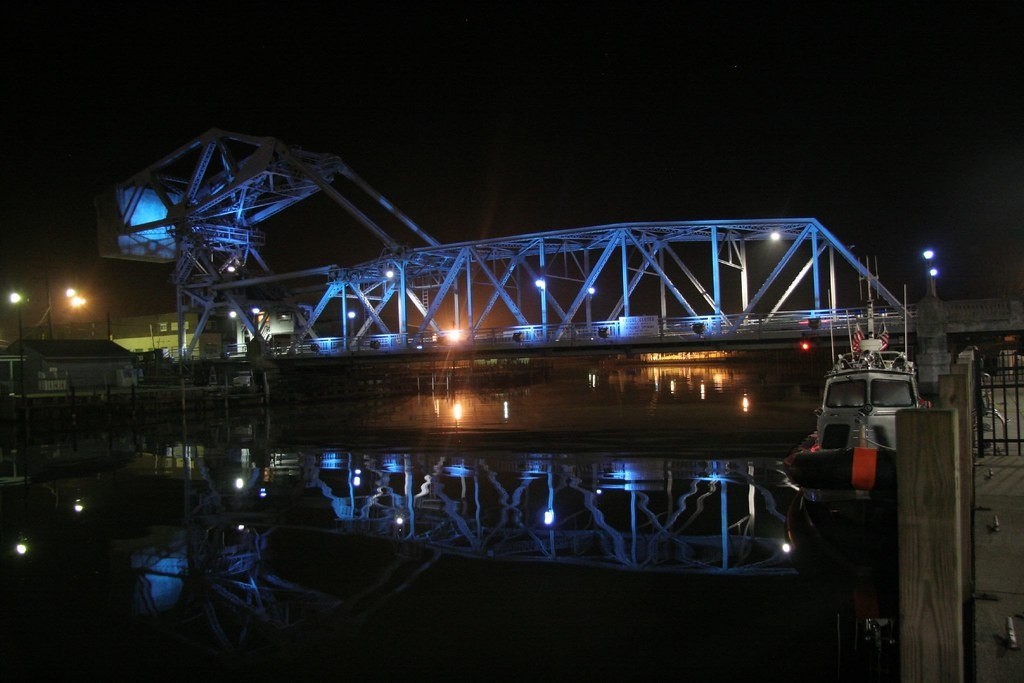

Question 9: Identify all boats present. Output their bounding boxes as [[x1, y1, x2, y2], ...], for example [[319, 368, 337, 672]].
[[785, 252, 933, 502], [0, 449, 134, 525]]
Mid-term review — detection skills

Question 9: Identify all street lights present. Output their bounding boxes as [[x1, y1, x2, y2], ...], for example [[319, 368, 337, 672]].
[[11, 294, 23, 396]]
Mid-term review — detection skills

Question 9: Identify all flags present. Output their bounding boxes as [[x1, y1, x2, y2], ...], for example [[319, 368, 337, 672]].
[[852, 321, 865, 352]]
